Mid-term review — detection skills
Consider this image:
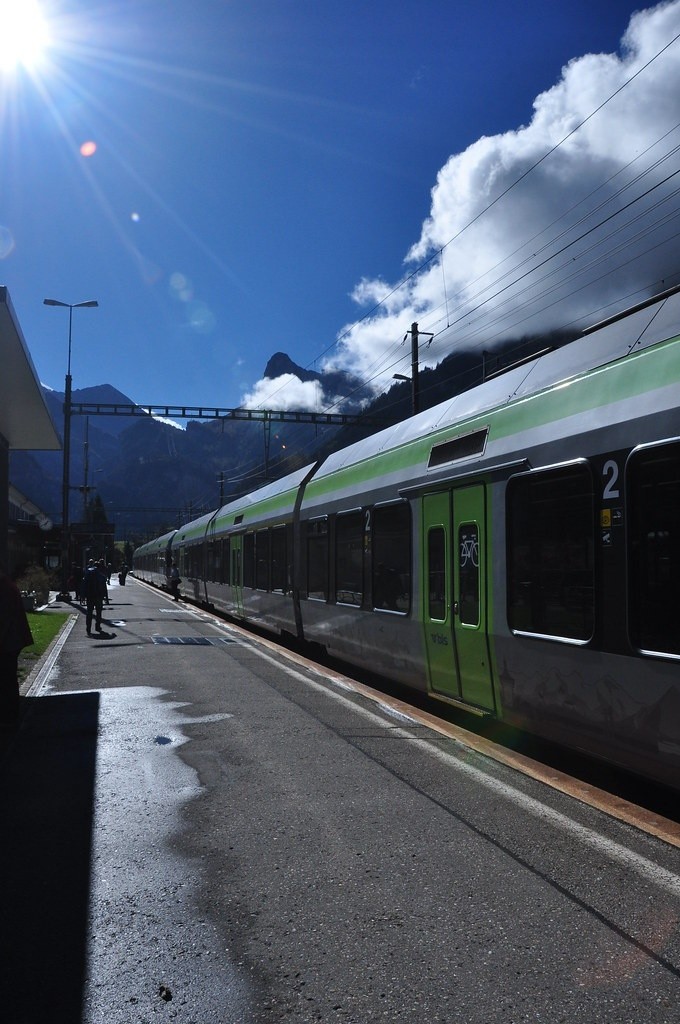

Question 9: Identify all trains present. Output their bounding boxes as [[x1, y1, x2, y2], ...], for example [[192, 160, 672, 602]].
[[132, 283, 678, 793]]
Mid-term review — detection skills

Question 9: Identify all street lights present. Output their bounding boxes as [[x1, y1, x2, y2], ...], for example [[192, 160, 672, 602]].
[[43, 298, 99, 374]]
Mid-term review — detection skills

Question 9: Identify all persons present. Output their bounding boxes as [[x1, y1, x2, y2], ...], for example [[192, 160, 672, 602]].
[[71, 558, 111, 637], [0, 560, 35, 728], [170, 563, 182, 601], [122, 561, 128, 585]]
